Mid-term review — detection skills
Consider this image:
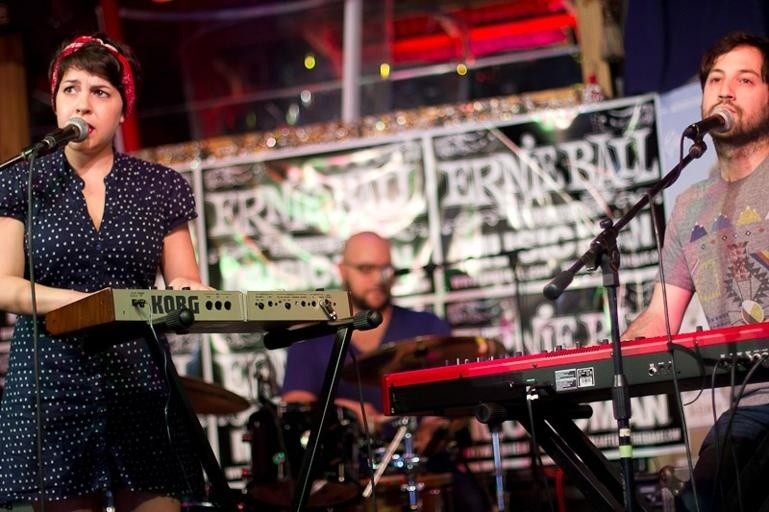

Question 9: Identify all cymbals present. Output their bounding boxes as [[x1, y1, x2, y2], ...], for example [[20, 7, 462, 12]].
[[179, 376, 250, 414], [342, 334, 507, 386]]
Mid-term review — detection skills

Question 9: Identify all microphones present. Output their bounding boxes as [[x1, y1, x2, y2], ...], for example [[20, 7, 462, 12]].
[[20, 115, 90, 160], [684, 108, 733, 137]]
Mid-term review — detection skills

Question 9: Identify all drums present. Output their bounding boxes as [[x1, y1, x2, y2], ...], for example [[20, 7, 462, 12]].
[[248, 399, 364, 512], [327, 473, 454, 512]]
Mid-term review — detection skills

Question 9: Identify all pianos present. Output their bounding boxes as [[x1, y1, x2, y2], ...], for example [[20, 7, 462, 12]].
[[380, 323, 769, 419], [45, 287, 354, 337]]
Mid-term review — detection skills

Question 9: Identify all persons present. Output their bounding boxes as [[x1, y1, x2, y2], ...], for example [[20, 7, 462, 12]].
[[0, 34, 216, 511], [282, 232, 474, 470], [618, 31, 769, 510]]
